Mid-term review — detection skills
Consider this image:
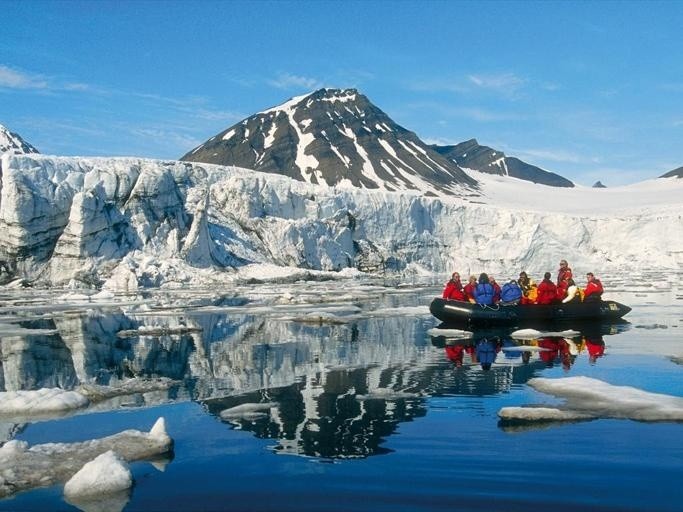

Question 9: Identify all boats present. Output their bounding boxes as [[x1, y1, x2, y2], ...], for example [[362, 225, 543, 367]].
[[431, 320, 632, 348], [429, 298, 633, 325]]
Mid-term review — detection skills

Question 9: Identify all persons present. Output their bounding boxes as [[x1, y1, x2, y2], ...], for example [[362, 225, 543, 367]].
[[445, 334, 605, 373], [443, 259, 604, 305]]
